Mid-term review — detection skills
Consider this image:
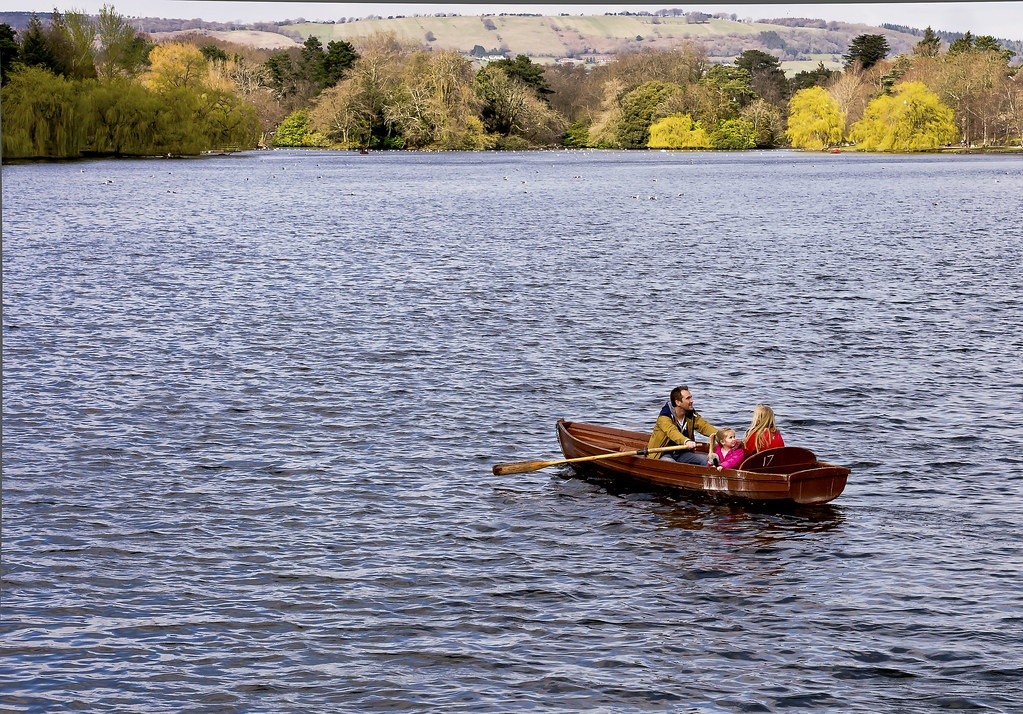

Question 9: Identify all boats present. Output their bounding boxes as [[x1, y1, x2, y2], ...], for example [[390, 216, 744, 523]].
[[554, 417, 852, 506], [360, 148, 368, 155]]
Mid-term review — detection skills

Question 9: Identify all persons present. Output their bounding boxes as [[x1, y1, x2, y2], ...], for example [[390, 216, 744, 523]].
[[644, 385, 722, 467], [740, 403, 786, 459], [707, 426, 746, 472]]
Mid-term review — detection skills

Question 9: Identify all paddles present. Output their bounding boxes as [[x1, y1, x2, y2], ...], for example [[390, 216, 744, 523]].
[[493, 442, 703, 476]]
